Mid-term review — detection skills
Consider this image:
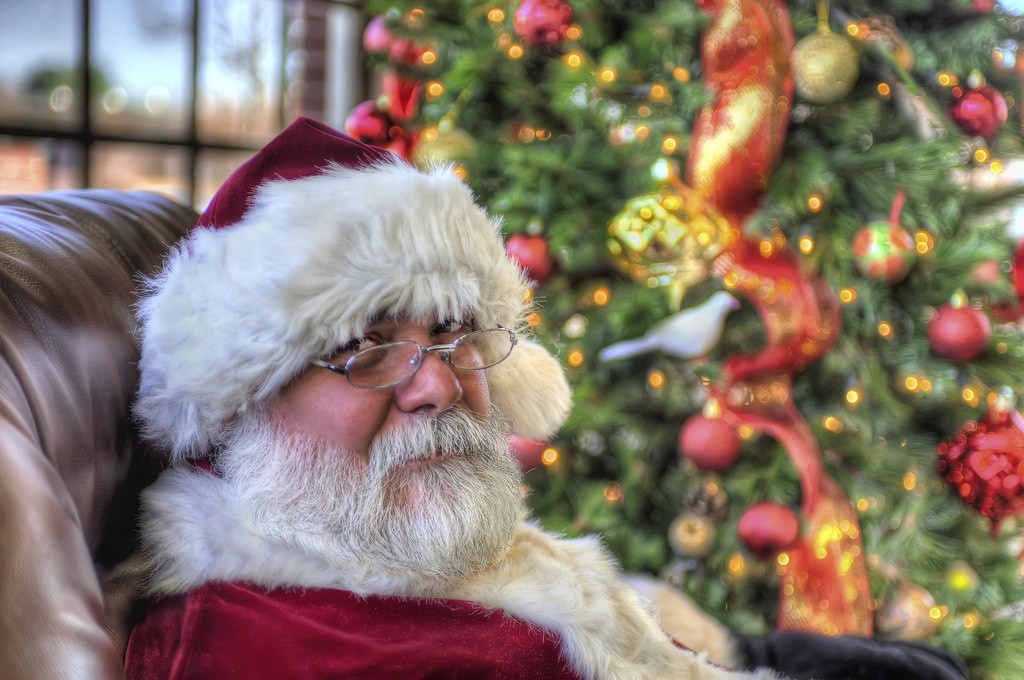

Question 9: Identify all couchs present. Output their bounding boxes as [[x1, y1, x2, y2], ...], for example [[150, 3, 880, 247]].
[[0, 187, 200, 680]]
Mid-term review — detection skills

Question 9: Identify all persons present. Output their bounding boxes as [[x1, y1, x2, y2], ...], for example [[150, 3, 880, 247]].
[[134, 114, 776, 680]]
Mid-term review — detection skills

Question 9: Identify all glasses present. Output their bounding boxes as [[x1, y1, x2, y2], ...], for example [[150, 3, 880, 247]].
[[307, 321, 518, 390]]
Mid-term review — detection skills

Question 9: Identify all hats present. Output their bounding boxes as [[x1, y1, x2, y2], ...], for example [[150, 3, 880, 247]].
[[132, 114, 574, 457]]
